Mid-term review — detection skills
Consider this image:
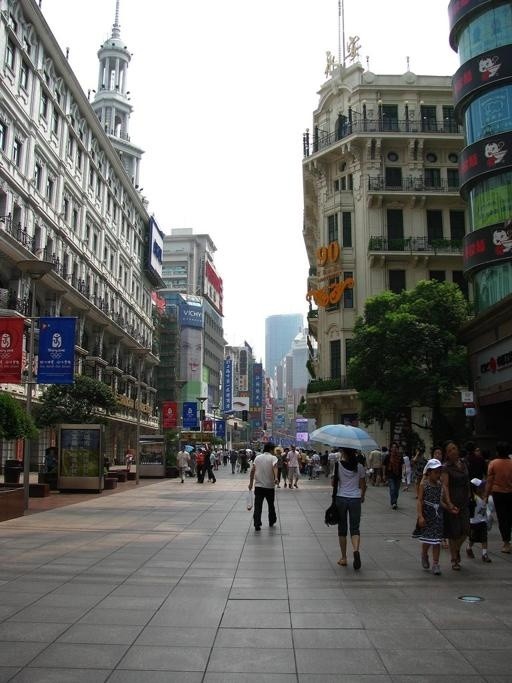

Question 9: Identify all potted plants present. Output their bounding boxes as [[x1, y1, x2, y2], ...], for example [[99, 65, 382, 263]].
[[165, 446, 180, 478], [0, 387, 43, 523]]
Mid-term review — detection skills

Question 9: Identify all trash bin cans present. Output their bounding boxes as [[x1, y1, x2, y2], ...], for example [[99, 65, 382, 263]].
[[4, 459, 20, 483]]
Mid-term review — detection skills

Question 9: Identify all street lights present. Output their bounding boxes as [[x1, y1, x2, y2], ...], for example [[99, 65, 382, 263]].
[[189, 397, 212, 447], [207, 405, 225, 450], [1, 259, 79, 508], [160, 379, 198, 477]]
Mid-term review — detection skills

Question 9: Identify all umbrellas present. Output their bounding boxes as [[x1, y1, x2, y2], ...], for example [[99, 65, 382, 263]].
[[308, 424, 380, 454]]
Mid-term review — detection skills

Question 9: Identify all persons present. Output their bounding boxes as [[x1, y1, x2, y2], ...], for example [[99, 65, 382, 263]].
[[331, 447, 366, 570], [274, 444, 334, 489], [180, 329, 204, 382], [126, 450, 133, 470], [364, 444, 428, 510], [177, 446, 261, 484], [249, 444, 279, 531], [410, 443, 511, 577]]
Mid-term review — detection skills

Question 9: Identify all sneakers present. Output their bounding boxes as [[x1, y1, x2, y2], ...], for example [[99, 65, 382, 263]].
[[181, 469, 219, 483], [255, 526, 261, 531], [422, 558, 430, 569], [466, 547, 475, 559], [499, 544, 512, 553], [482, 554, 491, 563], [352, 550, 362, 571], [277, 477, 319, 489], [451, 559, 462, 571], [371, 482, 410, 492], [432, 564, 441, 574], [269, 518, 277, 526], [391, 503, 399, 509], [232, 470, 248, 474], [338, 557, 348, 566]]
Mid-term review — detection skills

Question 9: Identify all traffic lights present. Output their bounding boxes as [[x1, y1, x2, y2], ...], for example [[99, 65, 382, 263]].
[[234, 421, 238, 430], [264, 422, 267, 430]]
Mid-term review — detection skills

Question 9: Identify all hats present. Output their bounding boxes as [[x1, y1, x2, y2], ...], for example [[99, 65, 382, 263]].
[[423, 458, 442, 474], [471, 477, 486, 489]]
